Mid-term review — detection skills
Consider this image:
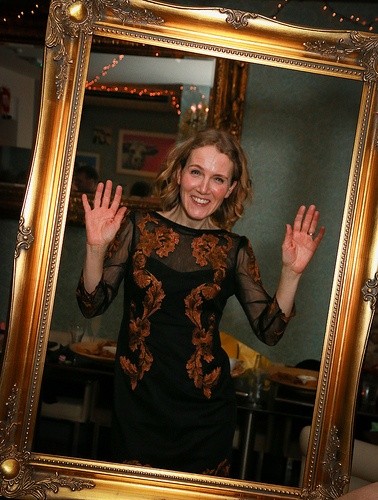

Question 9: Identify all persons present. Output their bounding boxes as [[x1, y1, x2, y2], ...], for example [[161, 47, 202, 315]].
[[77, 128, 326, 478]]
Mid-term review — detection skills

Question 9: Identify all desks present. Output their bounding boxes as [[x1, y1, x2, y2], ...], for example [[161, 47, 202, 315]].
[[43, 359, 378, 480]]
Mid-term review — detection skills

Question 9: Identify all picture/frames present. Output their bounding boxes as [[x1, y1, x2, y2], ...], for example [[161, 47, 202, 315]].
[[74, 149, 102, 178], [0, 0, 378, 500], [115, 130, 176, 178]]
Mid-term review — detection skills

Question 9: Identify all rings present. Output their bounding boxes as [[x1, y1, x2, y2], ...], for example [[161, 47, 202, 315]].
[[308, 233, 313, 236]]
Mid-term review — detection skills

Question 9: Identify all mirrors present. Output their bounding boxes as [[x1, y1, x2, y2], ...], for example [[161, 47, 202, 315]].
[[0, 16, 249, 229]]
